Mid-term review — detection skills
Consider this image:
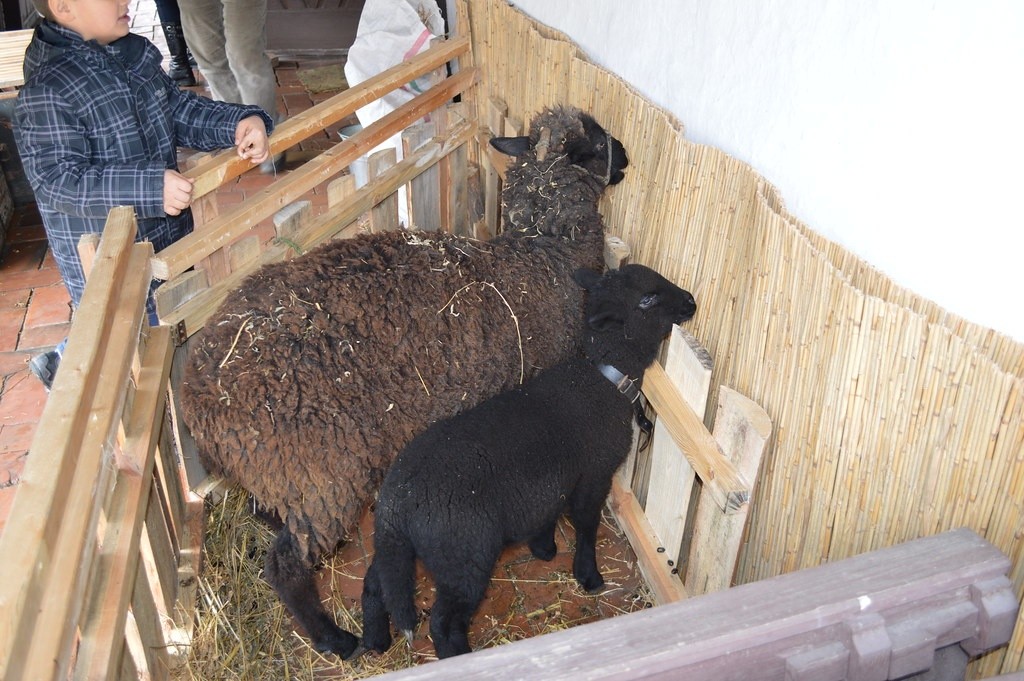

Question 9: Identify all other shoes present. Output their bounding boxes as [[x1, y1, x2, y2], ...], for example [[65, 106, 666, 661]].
[[260, 150, 286, 175], [30, 351, 61, 392]]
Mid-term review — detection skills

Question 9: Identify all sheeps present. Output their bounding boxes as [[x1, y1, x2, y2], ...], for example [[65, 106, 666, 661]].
[[172, 101, 699, 667]]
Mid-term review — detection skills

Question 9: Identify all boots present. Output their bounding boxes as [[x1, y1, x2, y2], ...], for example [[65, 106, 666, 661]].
[[160, 21, 196, 86]]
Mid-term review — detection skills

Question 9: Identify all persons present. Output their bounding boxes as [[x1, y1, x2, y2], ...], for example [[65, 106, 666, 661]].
[[153, 1, 288, 174], [1, 1, 317, 392]]
